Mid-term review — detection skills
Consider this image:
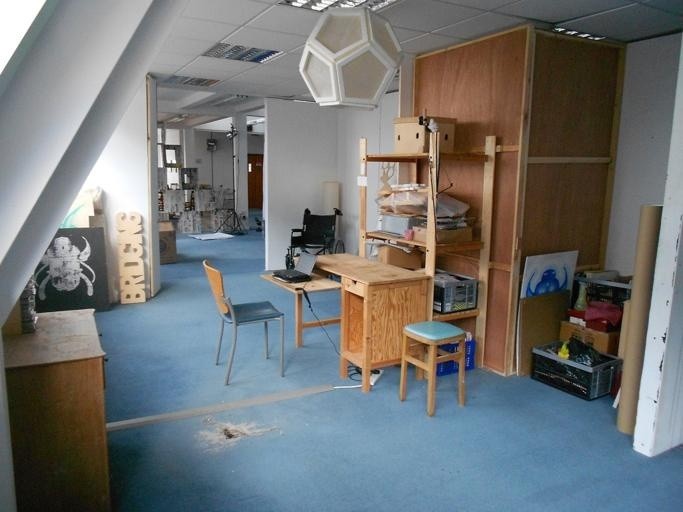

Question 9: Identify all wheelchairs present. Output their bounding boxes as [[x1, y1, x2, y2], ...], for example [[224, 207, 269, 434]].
[[286, 207, 345, 270]]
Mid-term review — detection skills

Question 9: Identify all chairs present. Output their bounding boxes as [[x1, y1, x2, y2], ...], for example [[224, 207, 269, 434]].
[[202, 259, 285, 385]]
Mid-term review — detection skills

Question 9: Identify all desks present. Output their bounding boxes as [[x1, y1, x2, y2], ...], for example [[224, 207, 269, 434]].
[[260, 253, 432, 393]]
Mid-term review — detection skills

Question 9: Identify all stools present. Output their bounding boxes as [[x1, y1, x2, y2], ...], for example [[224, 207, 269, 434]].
[[399, 321, 466, 416]]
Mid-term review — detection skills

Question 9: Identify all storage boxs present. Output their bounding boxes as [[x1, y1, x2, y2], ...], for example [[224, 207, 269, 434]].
[[559, 320, 620, 353], [393, 115, 458, 156], [159, 222, 176, 265], [377, 245, 422, 270], [413, 225, 473, 245]]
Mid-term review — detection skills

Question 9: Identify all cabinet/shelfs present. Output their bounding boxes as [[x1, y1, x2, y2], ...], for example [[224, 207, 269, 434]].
[[3, 308, 111, 512], [359, 137, 495, 367]]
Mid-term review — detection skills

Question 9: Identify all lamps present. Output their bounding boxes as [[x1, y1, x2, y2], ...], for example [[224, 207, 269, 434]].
[[299, 6, 404, 110]]
